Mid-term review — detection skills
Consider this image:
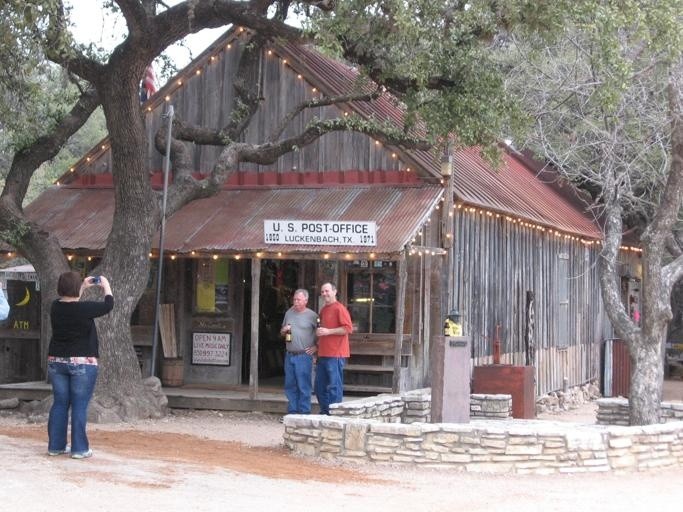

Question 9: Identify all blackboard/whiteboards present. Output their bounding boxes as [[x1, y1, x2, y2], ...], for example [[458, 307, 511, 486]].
[[191, 332, 232, 366]]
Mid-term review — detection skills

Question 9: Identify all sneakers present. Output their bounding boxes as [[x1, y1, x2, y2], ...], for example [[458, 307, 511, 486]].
[[279, 416, 283, 423], [48, 447, 71, 456], [72, 448, 94, 459]]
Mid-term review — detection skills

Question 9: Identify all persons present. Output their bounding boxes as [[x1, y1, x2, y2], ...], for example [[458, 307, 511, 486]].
[[46, 272, 115, 459], [630, 295, 641, 327], [280, 282, 353, 414], [0, 280, 10, 321]]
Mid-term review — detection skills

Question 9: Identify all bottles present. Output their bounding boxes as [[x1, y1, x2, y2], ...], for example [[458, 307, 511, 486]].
[[316, 318, 322, 329], [444, 318, 450, 336]]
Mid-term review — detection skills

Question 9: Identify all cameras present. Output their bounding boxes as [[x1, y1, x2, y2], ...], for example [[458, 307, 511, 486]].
[[91, 276, 101, 283]]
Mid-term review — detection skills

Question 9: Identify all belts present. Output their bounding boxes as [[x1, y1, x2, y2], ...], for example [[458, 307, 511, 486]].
[[287, 351, 305, 355]]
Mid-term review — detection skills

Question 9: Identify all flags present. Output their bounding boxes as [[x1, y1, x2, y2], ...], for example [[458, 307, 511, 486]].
[[140, 61, 157, 103]]
